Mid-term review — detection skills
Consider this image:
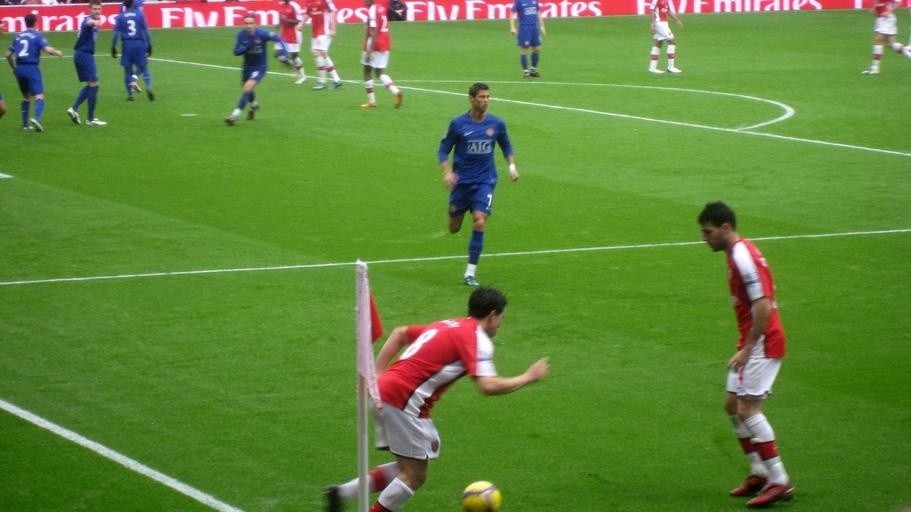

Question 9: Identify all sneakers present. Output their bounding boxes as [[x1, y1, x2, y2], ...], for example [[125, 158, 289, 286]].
[[862, 69, 880, 75], [246, 104, 259, 120], [66, 107, 81, 124], [648, 67, 682, 74], [23, 117, 44, 133], [128, 82, 154, 101], [224, 117, 238, 125], [393, 92, 402, 108], [463, 275, 481, 287], [361, 103, 377, 109], [523, 70, 541, 80], [85, 118, 107, 126], [729, 473, 768, 497], [745, 480, 796, 508], [291, 67, 343, 90], [322, 484, 345, 511]]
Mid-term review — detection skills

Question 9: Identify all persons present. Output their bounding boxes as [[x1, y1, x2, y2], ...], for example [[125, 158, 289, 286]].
[[861, 0, 911, 75], [508, 0, 546, 77], [322, 285, 549, 512], [1, 0, 156, 132], [696, 201, 796, 509], [437, 82, 519, 287], [224, 0, 408, 126], [649, 0, 683, 74]]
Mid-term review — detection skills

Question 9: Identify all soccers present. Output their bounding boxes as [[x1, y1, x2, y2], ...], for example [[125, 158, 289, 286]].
[[461, 480, 502, 512]]
